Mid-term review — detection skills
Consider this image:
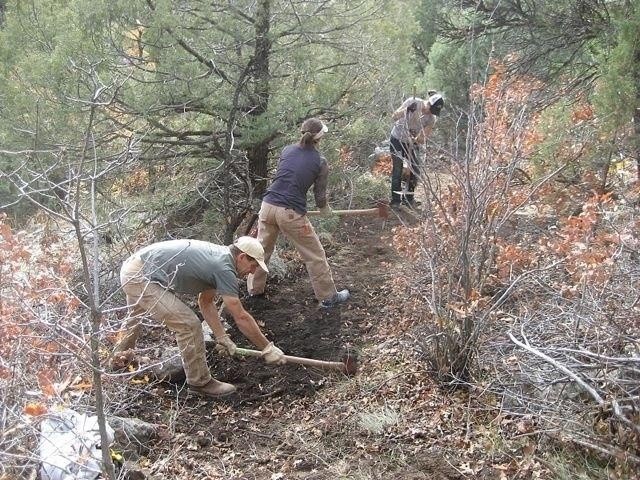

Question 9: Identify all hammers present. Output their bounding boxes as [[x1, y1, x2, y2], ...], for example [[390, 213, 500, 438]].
[[215, 344, 356, 375], [306, 203, 388, 218]]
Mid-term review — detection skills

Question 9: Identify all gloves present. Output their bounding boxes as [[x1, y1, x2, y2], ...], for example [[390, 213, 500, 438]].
[[320, 202, 334, 219], [406, 102, 416, 115], [214, 334, 245, 361], [260, 341, 288, 368]]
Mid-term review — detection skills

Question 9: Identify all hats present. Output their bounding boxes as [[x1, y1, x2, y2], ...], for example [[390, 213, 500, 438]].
[[233, 235, 269, 273], [312, 120, 328, 139], [429, 94, 444, 117]]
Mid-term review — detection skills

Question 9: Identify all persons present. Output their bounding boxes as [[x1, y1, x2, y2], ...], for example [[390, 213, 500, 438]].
[[107, 236, 287, 397], [249, 118, 350, 306], [389, 90, 444, 208]]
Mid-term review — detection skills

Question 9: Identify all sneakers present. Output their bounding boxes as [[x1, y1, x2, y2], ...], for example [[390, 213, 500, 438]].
[[186, 378, 237, 397], [390, 198, 423, 209], [109, 355, 151, 373], [321, 289, 351, 309]]
